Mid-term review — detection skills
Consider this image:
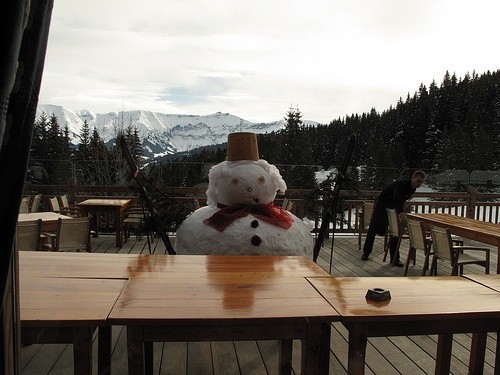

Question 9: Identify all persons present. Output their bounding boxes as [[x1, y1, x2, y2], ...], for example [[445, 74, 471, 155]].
[[360, 170, 426, 266]]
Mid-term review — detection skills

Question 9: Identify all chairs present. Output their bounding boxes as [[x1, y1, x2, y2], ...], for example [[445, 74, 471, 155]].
[[404, 217, 464, 277], [15, 193, 154, 254], [358, 201, 388, 251], [429, 223, 490, 276], [383, 208, 426, 266]]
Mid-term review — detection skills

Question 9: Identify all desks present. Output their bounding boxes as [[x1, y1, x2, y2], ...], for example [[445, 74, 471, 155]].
[[77, 198, 132, 247], [19, 250, 500, 375], [405, 213, 500, 274], [18, 213, 73, 220]]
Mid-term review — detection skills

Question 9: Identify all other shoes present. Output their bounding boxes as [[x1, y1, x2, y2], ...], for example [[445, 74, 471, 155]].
[[390, 258, 404, 267], [361, 253, 369, 260]]
